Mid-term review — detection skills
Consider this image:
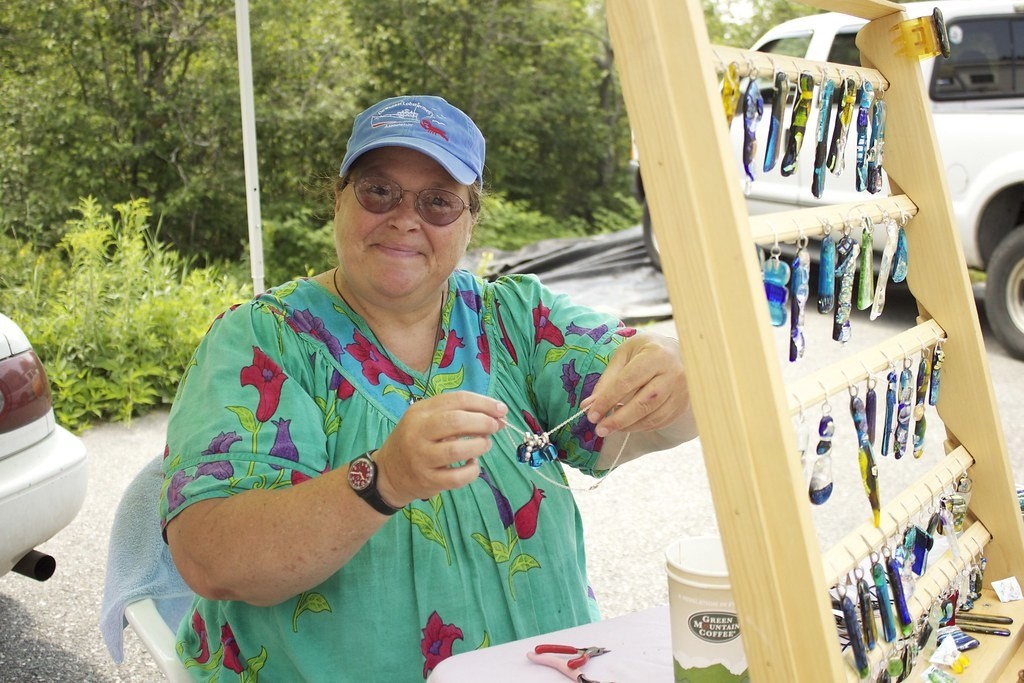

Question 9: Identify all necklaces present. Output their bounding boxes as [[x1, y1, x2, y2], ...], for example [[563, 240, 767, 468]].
[[492, 398, 631, 493], [332, 267, 448, 409]]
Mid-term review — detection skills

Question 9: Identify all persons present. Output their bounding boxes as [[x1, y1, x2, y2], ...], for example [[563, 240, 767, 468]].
[[159, 95, 701, 683]]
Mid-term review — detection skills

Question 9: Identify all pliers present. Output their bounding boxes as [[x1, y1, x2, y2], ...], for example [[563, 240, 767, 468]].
[[535, 644, 611, 670]]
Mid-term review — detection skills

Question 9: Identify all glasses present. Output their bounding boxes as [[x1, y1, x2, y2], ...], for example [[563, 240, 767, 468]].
[[343, 175, 474, 226]]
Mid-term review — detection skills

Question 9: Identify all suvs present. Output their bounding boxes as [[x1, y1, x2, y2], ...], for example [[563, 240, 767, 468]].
[[623, 3, 1022, 357]]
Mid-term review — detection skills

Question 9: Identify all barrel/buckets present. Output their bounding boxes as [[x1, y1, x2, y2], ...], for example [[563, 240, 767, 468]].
[[664, 531, 748, 682]]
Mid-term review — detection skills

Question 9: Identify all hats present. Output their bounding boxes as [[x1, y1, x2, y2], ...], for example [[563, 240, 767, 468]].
[[339, 96, 486, 195]]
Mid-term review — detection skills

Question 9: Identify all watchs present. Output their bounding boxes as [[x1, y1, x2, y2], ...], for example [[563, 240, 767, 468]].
[[345, 448, 408, 516]]
